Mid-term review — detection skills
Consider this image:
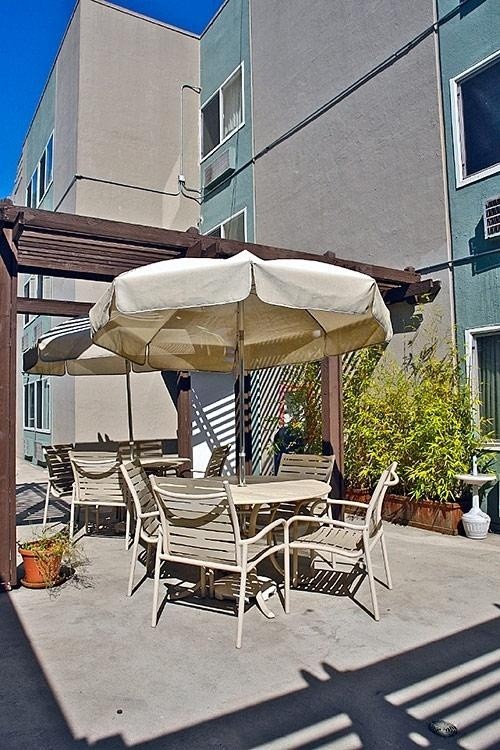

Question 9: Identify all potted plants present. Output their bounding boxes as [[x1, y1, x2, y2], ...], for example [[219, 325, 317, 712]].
[[16, 516, 94, 601]]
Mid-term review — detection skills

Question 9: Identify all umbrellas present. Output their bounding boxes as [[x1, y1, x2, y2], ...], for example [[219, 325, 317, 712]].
[[87, 250, 394, 487], [22, 315, 176, 458]]
